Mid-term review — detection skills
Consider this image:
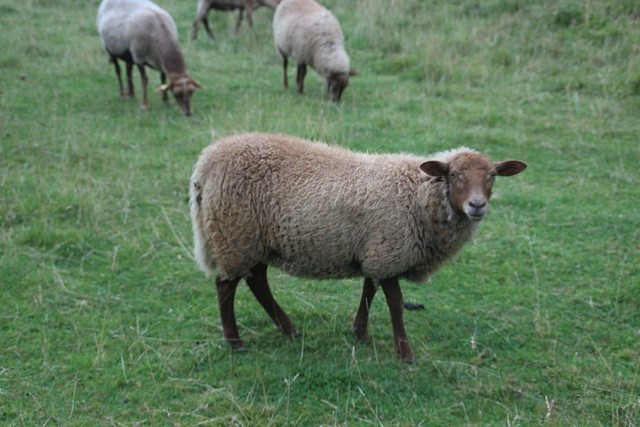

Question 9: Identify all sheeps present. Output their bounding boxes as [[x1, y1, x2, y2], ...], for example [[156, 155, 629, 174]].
[[96, 0, 204, 117], [190, 0, 280, 41], [271, 0, 360, 104], [187, 132, 528, 364]]
